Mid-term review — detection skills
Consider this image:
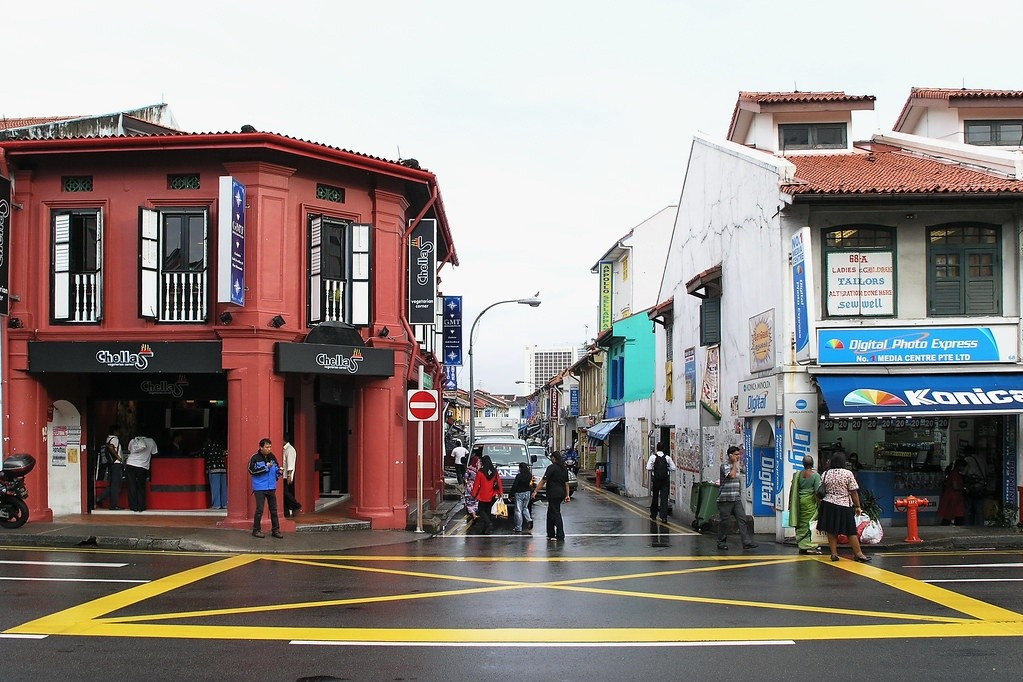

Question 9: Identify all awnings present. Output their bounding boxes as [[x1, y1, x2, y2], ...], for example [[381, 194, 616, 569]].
[[587, 421, 620, 440], [817, 375, 1023, 419]]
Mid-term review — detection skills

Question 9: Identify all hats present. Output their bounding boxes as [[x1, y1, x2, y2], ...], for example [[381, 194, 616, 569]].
[[549, 451, 561, 457]]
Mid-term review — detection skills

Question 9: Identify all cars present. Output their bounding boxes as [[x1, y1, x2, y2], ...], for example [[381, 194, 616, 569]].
[[518, 419, 579, 497]]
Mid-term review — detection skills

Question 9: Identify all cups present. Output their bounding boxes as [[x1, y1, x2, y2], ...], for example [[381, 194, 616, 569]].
[[844, 461, 851, 469]]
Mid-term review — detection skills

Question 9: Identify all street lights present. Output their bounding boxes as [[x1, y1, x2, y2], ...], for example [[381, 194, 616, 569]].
[[514, 380, 542, 446], [470, 297, 542, 449]]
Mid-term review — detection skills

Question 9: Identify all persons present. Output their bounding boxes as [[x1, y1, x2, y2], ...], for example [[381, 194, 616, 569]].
[[465, 456, 481, 520], [531, 452, 570, 540], [787, 455, 821, 555], [167, 430, 189, 456], [564, 446, 577, 459], [125, 423, 158, 511], [193, 433, 227, 509], [508, 463, 533, 531], [816, 452, 872, 562], [937, 445, 989, 525], [526, 435, 541, 445], [646, 442, 676, 523], [247, 439, 284, 538], [451, 441, 469, 485], [472, 455, 503, 534], [96, 425, 124, 510], [283, 432, 302, 517], [717, 446, 758, 550], [548, 436, 553, 453]]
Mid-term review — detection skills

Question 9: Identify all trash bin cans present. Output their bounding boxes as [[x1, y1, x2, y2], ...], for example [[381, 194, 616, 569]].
[[595, 462, 608, 479], [690, 481, 722, 532]]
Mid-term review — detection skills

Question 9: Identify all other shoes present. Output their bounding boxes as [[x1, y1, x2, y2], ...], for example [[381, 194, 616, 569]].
[[252, 531, 265, 538], [546, 536, 556, 540], [662, 518, 667, 522], [513, 527, 522, 532], [718, 546, 728, 550], [96, 496, 106, 507], [528, 521, 533, 530], [831, 555, 839, 561], [109, 505, 124, 510], [650, 515, 656, 520], [271, 531, 283, 538], [743, 543, 758, 549], [949, 521, 956, 528], [857, 556, 872, 563]]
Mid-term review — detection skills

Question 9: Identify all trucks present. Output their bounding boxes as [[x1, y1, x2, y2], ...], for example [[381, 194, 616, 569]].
[[473, 417, 519, 453]]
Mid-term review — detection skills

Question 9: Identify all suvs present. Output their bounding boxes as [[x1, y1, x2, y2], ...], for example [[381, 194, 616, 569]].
[[460, 439, 538, 513]]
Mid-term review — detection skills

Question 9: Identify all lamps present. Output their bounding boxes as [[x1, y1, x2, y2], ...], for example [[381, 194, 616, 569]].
[[378, 326, 389, 337], [219, 312, 232, 325], [9, 318, 23, 328], [272, 315, 286, 328]]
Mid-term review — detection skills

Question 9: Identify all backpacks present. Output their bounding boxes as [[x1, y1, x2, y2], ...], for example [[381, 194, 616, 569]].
[[100, 436, 119, 466], [654, 452, 668, 486]]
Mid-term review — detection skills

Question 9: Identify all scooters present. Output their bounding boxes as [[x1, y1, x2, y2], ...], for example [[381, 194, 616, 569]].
[[0, 452, 36, 529]]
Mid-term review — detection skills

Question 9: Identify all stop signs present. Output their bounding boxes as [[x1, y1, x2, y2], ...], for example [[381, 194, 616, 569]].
[[409, 390, 438, 420]]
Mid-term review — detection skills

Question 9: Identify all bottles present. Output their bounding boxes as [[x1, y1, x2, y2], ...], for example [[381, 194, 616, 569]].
[[873, 439, 934, 471]]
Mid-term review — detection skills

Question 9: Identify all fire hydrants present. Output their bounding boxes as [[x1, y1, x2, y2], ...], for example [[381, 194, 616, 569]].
[[596, 469, 601, 489]]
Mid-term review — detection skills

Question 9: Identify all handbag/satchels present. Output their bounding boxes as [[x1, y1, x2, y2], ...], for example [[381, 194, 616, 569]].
[[856, 513, 884, 544], [128, 436, 147, 453], [491, 497, 507, 520], [816, 470, 827, 499]]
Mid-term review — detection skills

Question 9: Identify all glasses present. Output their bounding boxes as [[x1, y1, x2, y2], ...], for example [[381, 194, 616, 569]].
[[732, 454, 740, 456]]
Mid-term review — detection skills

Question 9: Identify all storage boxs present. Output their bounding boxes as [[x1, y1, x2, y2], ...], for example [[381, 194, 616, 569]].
[[2, 454, 36, 476]]
